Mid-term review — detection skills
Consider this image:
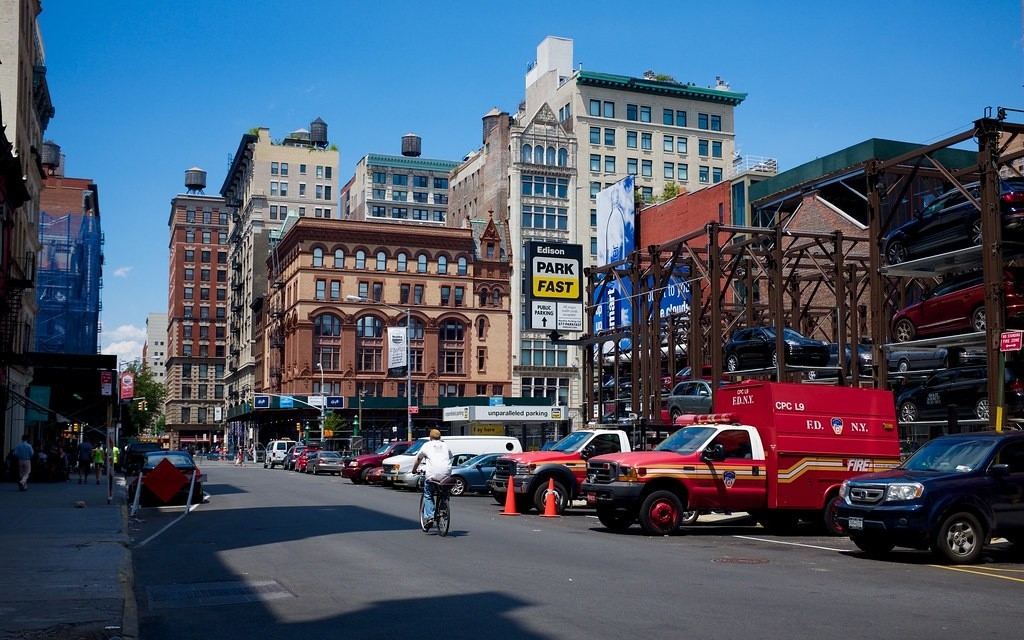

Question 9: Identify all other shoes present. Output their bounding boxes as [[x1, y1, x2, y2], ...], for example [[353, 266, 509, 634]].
[[443, 513, 448, 519], [424, 521, 434, 529], [79, 479, 82, 484], [20, 488, 26, 491], [84, 480, 87, 482], [96, 481, 100, 485], [18, 482, 23, 490]]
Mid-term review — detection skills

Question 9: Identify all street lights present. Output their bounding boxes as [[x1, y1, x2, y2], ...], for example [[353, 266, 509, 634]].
[[115, 356, 160, 450], [346, 295, 413, 446], [316, 363, 325, 442]]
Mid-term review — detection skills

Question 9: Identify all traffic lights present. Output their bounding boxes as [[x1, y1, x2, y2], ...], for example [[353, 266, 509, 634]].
[[248, 396, 253, 411], [144, 402, 147, 411], [296, 423, 300, 431], [137, 402, 143, 410]]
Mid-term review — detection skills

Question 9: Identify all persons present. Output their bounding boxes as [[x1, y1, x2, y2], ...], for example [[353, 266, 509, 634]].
[[235, 452, 243, 466], [14, 434, 120, 491], [187, 444, 194, 457], [413, 429, 453, 529]]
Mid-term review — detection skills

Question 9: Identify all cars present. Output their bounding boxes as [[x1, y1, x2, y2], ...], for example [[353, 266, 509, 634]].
[[261, 435, 522, 496], [593, 364, 731, 425], [123, 441, 204, 504]]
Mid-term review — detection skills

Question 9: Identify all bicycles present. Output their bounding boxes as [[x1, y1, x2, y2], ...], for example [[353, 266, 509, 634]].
[[411, 468, 455, 538]]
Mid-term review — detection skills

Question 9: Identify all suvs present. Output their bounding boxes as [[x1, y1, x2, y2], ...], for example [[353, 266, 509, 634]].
[[808, 342, 988, 379], [724, 326, 830, 374], [883, 177, 1024, 267], [888, 266, 1024, 344], [896, 363, 1024, 424], [837, 428, 1024, 564]]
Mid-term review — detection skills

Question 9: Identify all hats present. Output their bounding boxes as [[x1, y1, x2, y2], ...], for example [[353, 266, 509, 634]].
[[429, 429, 441, 438]]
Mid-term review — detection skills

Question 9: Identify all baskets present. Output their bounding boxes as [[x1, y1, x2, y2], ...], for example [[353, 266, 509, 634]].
[[419, 477, 426, 488]]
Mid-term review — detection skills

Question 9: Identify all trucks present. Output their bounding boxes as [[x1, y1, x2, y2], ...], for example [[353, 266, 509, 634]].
[[583, 382, 903, 537], [484, 429, 639, 518]]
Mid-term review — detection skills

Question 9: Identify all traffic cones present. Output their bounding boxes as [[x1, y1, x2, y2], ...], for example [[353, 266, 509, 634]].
[[498, 474, 521, 516], [539, 476, 561, 517]]
